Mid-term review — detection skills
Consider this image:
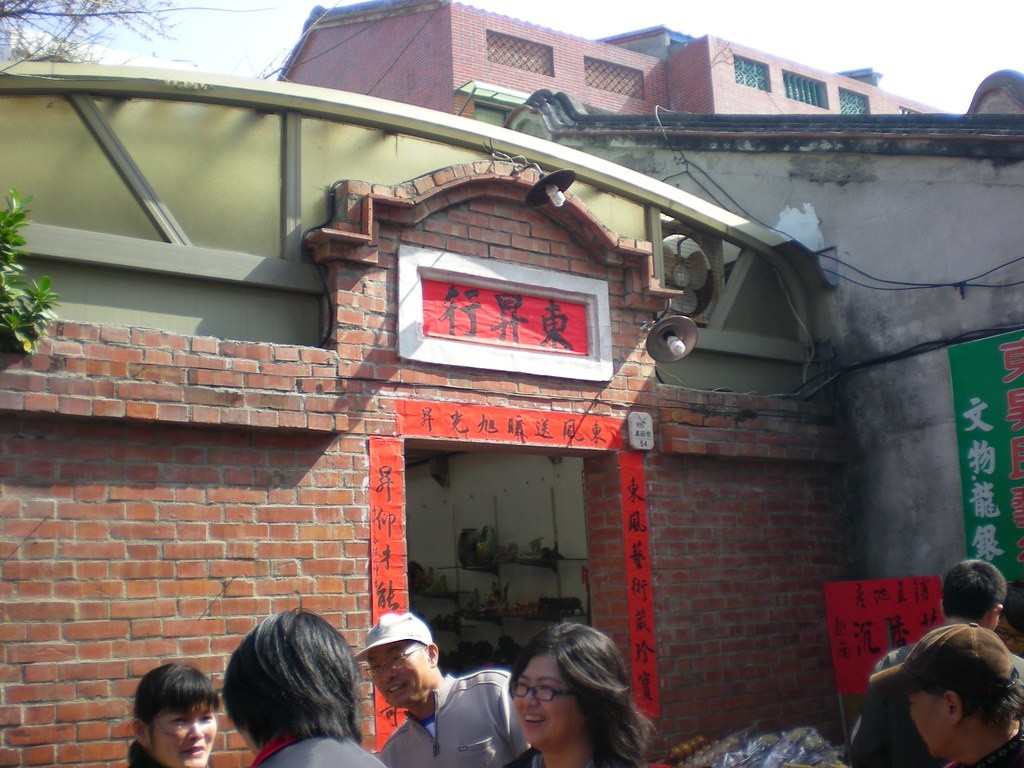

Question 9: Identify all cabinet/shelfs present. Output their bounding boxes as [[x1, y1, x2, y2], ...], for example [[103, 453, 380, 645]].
[[409, 558, 588, 636]]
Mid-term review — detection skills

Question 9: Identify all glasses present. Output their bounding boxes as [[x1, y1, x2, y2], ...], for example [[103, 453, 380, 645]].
[[364, 646, 427, 678], [993, 629, 1024, 643], [513, 681, 577, 702]]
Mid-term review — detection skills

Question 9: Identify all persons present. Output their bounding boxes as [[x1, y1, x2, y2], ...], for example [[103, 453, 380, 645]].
[[501, 622, 654, 768], [126, 664, 220, 768], [849, 559, 1024, 768], [222, 608, 388, 768], [354, 613, 529, 768]]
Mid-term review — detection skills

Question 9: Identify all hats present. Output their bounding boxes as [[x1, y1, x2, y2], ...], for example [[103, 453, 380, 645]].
[[869, 622, 1019, 702], [353, 612, 432, 661]]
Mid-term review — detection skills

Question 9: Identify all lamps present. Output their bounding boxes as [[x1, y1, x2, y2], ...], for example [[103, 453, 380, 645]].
[[639, 297, 698, 365], [511, 162, 576, 207]]
